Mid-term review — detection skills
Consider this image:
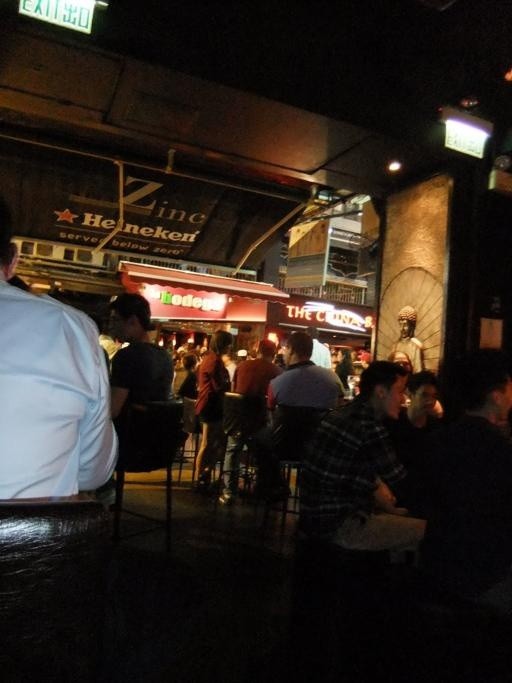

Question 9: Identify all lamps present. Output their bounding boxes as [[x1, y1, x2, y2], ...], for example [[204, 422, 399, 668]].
[[441, 106, 494, 160]]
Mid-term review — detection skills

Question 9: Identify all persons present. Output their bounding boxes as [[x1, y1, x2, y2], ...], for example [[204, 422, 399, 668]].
[[111, 294, 510, 560], [1, 198, 118, 505]]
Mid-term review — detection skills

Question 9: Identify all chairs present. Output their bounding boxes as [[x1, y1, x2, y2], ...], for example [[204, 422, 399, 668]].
[[108, 396, 337, 553], [0, 502, 109, 682]]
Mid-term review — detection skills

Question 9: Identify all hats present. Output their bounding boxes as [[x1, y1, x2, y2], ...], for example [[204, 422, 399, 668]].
[[107, 294, 156, 332]]
